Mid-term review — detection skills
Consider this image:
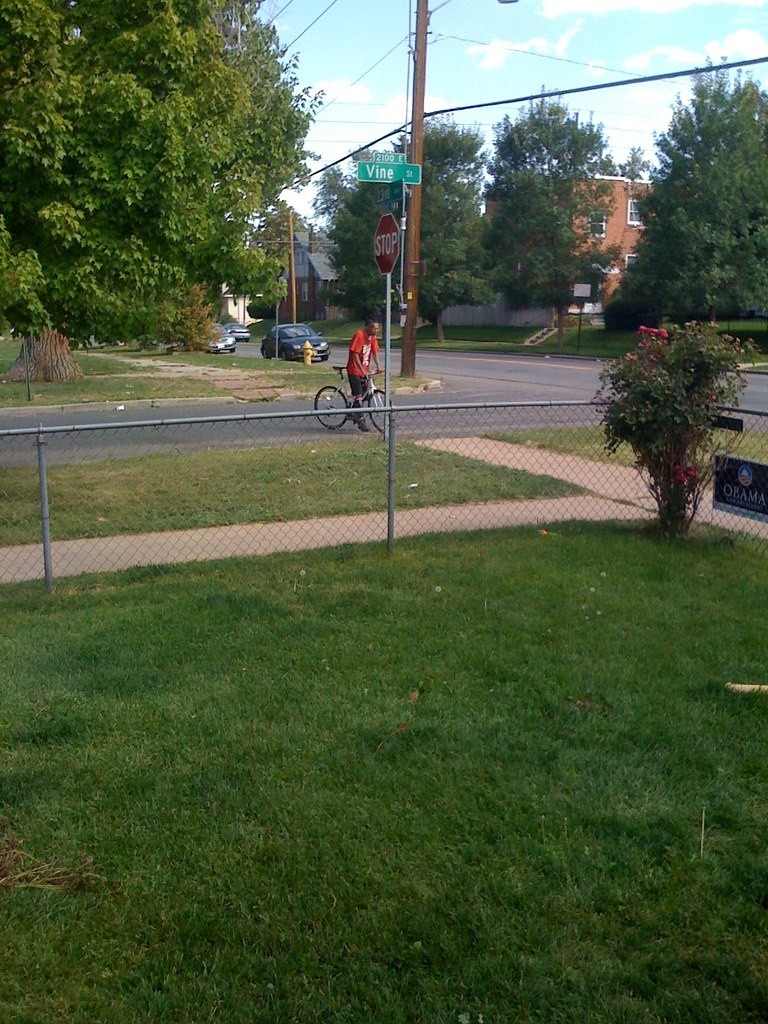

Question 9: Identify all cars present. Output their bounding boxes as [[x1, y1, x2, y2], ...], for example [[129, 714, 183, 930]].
[[204, 330, 238, 353], [224, 323, 250, 342], [260, 324, 332, 361]]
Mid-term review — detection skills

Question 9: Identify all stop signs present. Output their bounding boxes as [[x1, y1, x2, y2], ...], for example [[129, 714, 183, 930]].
[[373, 213, 403, 277]]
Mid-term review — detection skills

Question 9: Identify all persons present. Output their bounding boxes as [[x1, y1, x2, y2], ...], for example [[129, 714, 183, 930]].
[[347, 319, 383, 432]]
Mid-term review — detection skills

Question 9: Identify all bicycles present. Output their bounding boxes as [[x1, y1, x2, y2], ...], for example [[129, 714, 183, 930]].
[[313, 366, 394, 433]]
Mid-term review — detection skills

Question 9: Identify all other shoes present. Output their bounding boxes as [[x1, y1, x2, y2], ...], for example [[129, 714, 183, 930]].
[[359, 422, 368, 431]]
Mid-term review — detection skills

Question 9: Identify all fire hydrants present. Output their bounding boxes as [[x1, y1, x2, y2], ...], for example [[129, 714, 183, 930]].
[[301, 340, 315, 365]]
